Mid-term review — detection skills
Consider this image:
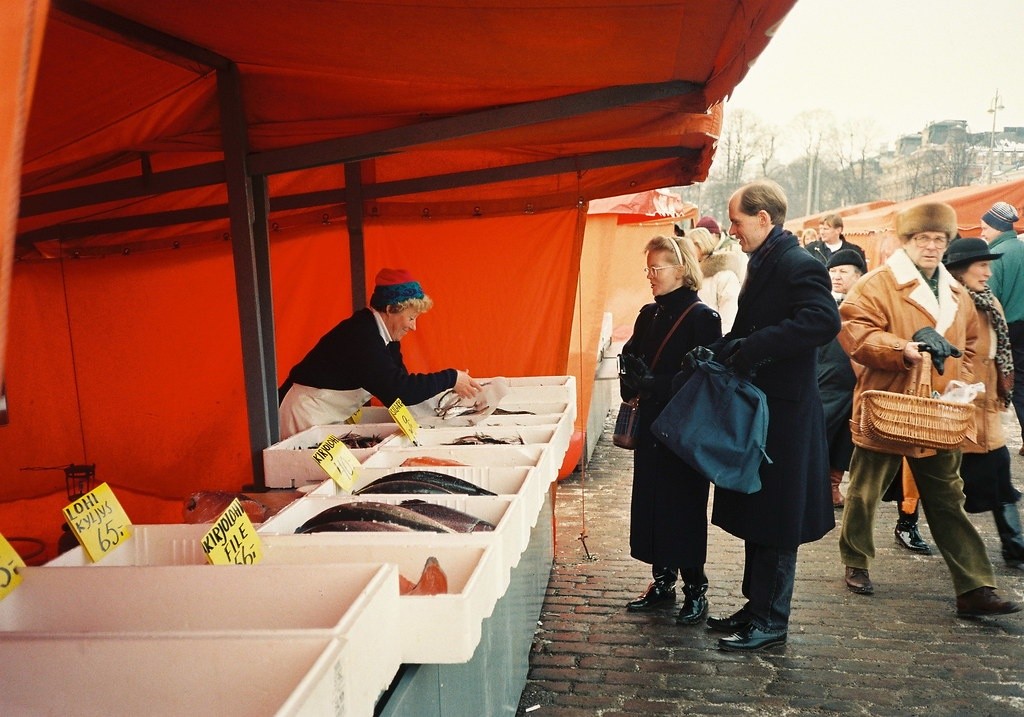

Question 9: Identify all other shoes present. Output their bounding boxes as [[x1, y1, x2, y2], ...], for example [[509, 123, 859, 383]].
[[1019, 447, 1024, 456], [830, 470, 845, 507]]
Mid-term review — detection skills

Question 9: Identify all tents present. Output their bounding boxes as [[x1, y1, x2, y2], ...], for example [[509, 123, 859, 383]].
[[556, 176, 1024, 565]]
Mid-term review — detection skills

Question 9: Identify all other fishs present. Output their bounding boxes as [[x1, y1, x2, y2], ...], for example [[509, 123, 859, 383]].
[[295, 376, 537, 600]]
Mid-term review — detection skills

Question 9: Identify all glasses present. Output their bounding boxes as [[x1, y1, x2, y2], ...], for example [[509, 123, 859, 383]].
[[644, 265, 674, 277], [911, 234, 949, 249]]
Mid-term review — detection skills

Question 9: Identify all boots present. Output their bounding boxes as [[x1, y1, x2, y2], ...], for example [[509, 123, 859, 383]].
[[993, 504, 1024, 567]]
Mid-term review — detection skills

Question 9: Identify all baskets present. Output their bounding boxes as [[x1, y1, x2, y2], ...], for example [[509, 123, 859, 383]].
[[859, 351, 976, 451]]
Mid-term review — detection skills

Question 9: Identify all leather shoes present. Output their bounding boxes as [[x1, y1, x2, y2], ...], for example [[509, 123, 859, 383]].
[[676, 596, 708, 624], [956, 587, 1022, 614], [627, 583, 676, 612], [706, 610, 751, 632], [895, 521, 931, 554], [719, 622, 787, 652], [845, 566, 874, 594]]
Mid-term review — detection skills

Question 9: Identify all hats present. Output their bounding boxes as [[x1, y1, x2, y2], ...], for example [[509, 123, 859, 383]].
[[372, 268, 423, 307], [827, 249, 864, 269], [895, 204, 958, 242], [697, 216, 720, 234], [982, 202, 1019, 231], [945, 238, 1004, 266]]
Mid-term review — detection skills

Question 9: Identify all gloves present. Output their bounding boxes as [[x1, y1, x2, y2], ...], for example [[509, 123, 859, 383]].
[[619, 354, 648, 391], [680, 346, 714, 370], [722, 349, 754, 383], [913, 326, 962, 375]]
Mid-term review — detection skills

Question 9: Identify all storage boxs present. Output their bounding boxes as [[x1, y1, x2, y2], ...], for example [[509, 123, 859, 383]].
[[0, 375, 576, 717]]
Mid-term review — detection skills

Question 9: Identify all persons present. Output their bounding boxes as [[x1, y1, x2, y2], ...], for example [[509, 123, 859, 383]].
[[834, 203, 1024, 618], [618, 235, 723, 626], [683, 180, 842, 650], [278, 268, 482, 440]]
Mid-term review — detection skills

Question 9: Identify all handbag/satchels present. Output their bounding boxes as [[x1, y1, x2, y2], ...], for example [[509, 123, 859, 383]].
[[650, 361, 770, 493], [613, 402, 639, 449]]
